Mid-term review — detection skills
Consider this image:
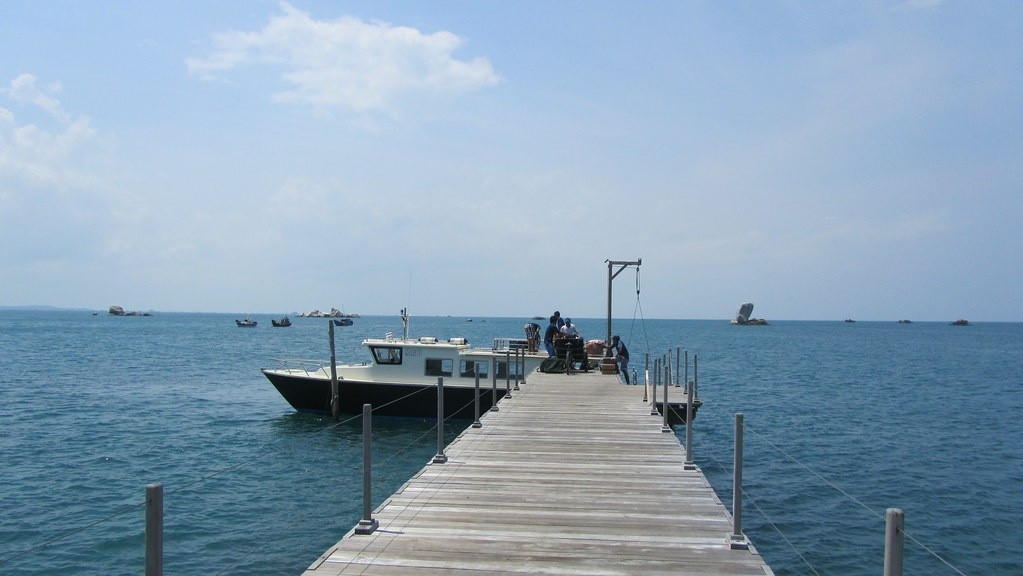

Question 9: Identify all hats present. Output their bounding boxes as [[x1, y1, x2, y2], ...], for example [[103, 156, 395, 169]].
[[565, 318, 571, 323], [610, 335, 620, 339]]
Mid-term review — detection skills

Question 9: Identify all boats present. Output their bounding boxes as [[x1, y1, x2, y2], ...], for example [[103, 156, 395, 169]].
[[844, 318, 855, 323], [334, 318, 354, 326], [261, 306, 704, 429], [271, 316, 292, 327], [235, 319, 258, 328]]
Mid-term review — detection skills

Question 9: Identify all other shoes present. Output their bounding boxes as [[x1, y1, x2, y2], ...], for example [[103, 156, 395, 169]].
[[615, 371, 620, 374]]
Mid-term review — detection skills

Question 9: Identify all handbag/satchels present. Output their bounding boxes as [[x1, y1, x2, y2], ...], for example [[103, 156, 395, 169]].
[[537, 354, 566, 374]]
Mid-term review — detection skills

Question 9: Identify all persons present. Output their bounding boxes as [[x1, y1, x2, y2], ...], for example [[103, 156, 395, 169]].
[[544, 316, 560, 357], [281, 317, 289, 324], [560, 318, 578, 338], [601, 335, 629, 385], [524, 323, 541, 352], [554, 311, 565, 330]]
